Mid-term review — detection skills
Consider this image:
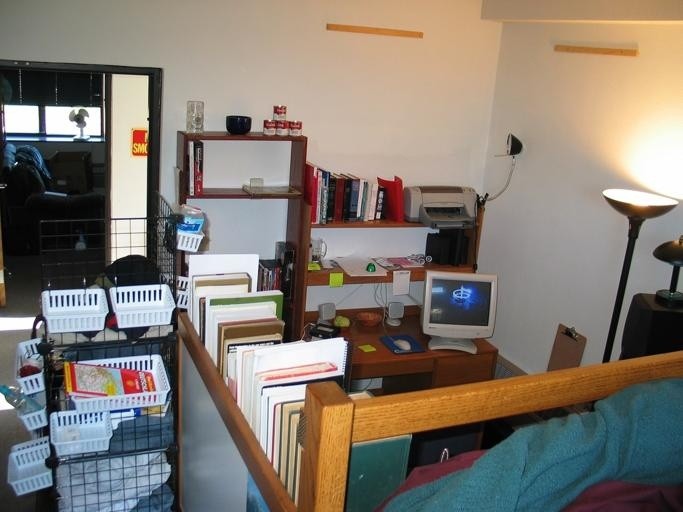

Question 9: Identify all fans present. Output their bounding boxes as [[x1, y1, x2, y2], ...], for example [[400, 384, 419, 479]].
[[70, 109, 90, 141]]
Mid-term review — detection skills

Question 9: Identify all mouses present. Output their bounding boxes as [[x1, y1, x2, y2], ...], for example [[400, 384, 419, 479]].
[[393, 339, 411, 350]]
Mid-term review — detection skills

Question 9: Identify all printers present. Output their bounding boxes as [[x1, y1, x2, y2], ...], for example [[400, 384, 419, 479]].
[[404, 184, 478, 230]]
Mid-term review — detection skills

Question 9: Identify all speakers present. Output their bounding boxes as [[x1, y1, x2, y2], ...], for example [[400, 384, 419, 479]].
[[386, 302, 405, 326], [315, 303, 336, 325]]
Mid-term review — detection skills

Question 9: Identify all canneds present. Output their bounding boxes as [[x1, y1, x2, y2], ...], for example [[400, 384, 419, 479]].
[[276, 121, 289, 136], [289, 122, 302, 136], [273, 106, 286, 121], [263, 120, 275, 136]]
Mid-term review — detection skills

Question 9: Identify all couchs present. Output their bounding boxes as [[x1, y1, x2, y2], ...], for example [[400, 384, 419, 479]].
[[3, 144, 104, 256]]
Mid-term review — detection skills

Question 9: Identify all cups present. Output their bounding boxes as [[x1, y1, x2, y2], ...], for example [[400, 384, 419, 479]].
[[311, 238, 328, 263], [187, 100, 204, 134]]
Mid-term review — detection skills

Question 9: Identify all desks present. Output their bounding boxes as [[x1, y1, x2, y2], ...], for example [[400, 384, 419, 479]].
[[305, 197, 498, 450]]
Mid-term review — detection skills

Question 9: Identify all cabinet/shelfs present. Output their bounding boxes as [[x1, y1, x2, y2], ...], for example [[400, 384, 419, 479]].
[[174, 131, 307, 341]]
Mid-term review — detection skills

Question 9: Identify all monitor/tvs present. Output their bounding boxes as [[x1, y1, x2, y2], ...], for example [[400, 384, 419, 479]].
[[421, 269, 497, 355]]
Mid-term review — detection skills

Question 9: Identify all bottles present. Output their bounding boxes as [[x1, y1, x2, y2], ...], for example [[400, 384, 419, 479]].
[[0, 386, 40, 414]]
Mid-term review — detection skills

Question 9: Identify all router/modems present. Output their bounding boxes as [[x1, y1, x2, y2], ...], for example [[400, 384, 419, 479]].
[[309, 324, 341, 339]]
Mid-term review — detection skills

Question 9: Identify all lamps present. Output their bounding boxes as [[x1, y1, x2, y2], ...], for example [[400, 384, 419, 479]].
[[482, 133, 522, 206], [653, 236, 683, 309], [590, 189, 679, 412]]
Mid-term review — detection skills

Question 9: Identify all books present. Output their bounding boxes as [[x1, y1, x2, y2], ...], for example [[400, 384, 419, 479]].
[[63, 361, 157, 401], [306, 161, 405, 224], [193, 272, 412, 506], [242, 185, 303, 198], [334, 254, 389, 279]]
